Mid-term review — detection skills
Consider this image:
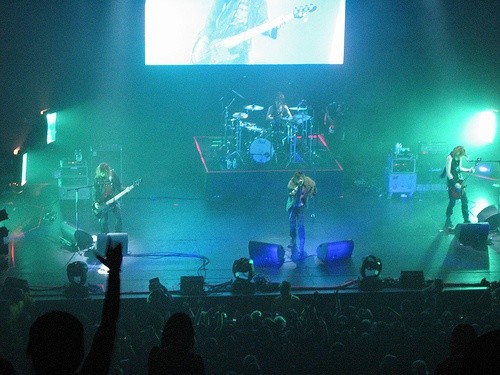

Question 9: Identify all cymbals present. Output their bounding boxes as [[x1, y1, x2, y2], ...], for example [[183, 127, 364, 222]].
[[232, 112, 248, 119], [289, 106, 307, 111], [242, 105, 265, 111]]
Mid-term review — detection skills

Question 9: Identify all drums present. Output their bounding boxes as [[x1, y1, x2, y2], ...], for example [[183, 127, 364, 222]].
[[291, 113, 312, 126], [241, 127, 267, 148], [247, 136, 275, 164]]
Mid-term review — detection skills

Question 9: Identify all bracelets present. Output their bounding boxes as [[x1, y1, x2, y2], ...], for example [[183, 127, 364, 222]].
[[108, 268, 121, 273], [451, 179, 456, 186]]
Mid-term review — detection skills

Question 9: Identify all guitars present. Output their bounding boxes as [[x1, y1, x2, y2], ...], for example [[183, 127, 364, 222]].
[[90, 177, 143, 220], [449, 157, 482, 199]]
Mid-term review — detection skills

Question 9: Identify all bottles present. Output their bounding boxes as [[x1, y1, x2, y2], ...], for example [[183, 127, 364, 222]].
[[396, 142, 402, 154]]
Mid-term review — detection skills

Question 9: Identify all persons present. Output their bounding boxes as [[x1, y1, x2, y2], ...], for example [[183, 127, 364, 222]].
[[0, 236, 500, 375], [191, 0, 295, 64], [444, 146, 475, 227], [286, 171, 316, 257], [266, 94, 292, 130], [93, 162, 128, 233]]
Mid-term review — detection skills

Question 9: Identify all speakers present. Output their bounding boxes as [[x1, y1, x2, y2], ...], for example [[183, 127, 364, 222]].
[[249, 240, 284, 264], [456, 222, 490, 242], [477, 205, 500, 231], [60, 222, 92, 249], [97, 232, 129, 258], [318, 240, 354, 263]]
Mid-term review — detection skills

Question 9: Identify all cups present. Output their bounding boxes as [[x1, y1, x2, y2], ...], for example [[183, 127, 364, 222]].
[[76, 153, 82, 161]]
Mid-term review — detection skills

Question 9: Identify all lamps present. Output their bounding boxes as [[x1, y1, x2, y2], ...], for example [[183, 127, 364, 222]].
[[232, 257, 257, 295], [65, 261, 90, 298], [362, 255, 383, 291]]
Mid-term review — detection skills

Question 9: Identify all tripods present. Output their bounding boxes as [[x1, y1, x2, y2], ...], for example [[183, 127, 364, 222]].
[[214, 97, 322, 168], [66, 185, 96, 265]]
